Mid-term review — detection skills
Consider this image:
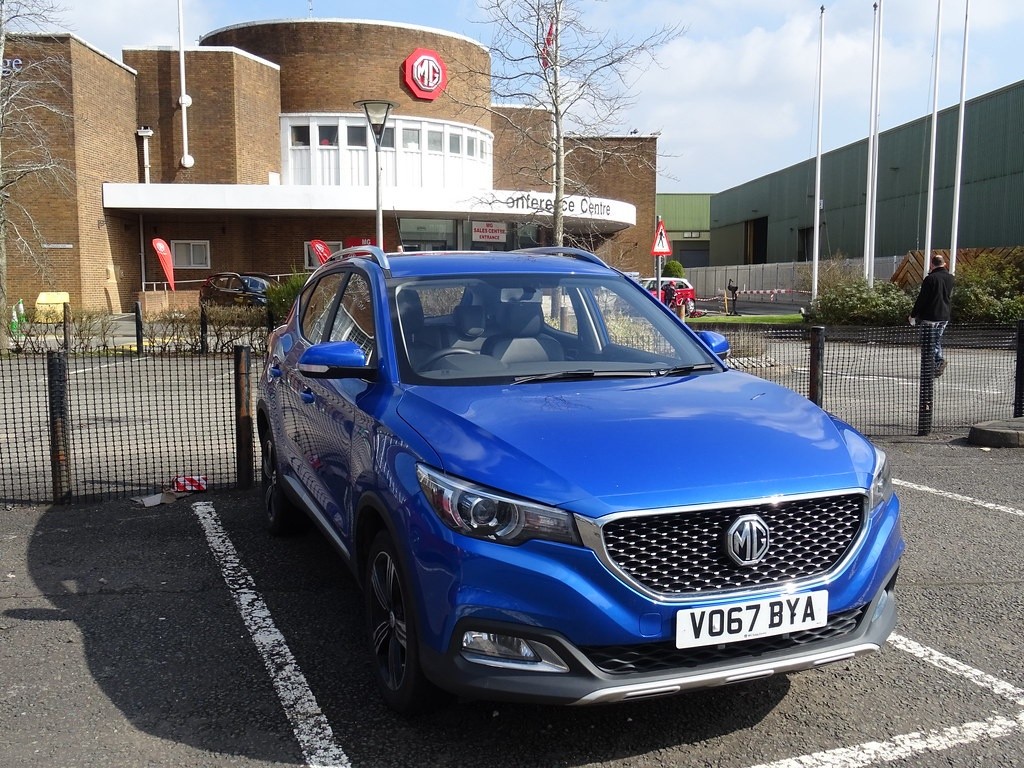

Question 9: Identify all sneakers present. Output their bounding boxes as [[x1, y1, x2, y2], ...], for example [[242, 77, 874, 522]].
[[937, 360, 947, 377]]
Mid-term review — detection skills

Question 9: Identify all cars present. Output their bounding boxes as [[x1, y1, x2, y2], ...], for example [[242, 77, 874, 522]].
[[639, 278, 695, 312]]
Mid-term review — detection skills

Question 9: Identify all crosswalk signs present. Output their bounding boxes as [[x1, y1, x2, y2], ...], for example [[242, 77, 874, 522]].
[[650, 221, 672, 255]]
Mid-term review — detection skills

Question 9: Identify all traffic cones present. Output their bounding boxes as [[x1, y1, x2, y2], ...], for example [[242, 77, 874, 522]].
[[9, 305, 21, 332], [17, 299, 27, 322]]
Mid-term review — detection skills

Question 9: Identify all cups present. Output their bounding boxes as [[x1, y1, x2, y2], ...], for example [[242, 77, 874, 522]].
[[910, 318, 915, 325]]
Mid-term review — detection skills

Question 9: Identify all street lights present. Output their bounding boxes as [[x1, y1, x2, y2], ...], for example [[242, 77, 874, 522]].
[[353, 98, 401, 253]]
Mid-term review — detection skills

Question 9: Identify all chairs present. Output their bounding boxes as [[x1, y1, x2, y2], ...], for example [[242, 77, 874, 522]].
[[443, 305, 494, 356], [480, 302, 565, 362], [386, 287, 442, 369]]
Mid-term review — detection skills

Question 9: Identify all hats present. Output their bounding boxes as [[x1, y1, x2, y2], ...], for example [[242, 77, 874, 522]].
[[670, 281, 676, 286]]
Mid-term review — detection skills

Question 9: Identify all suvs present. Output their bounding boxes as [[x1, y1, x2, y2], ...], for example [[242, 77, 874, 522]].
[[255, 245, 906, 715], [199, 272, 283, 307]]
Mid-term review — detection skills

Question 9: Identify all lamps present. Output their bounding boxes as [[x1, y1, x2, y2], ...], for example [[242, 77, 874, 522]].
[[630, 129, 638, 135]]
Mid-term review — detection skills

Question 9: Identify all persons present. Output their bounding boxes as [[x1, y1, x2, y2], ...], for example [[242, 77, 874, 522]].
[[664, 281, 675, 308], [909, 255, 955, 379]]
